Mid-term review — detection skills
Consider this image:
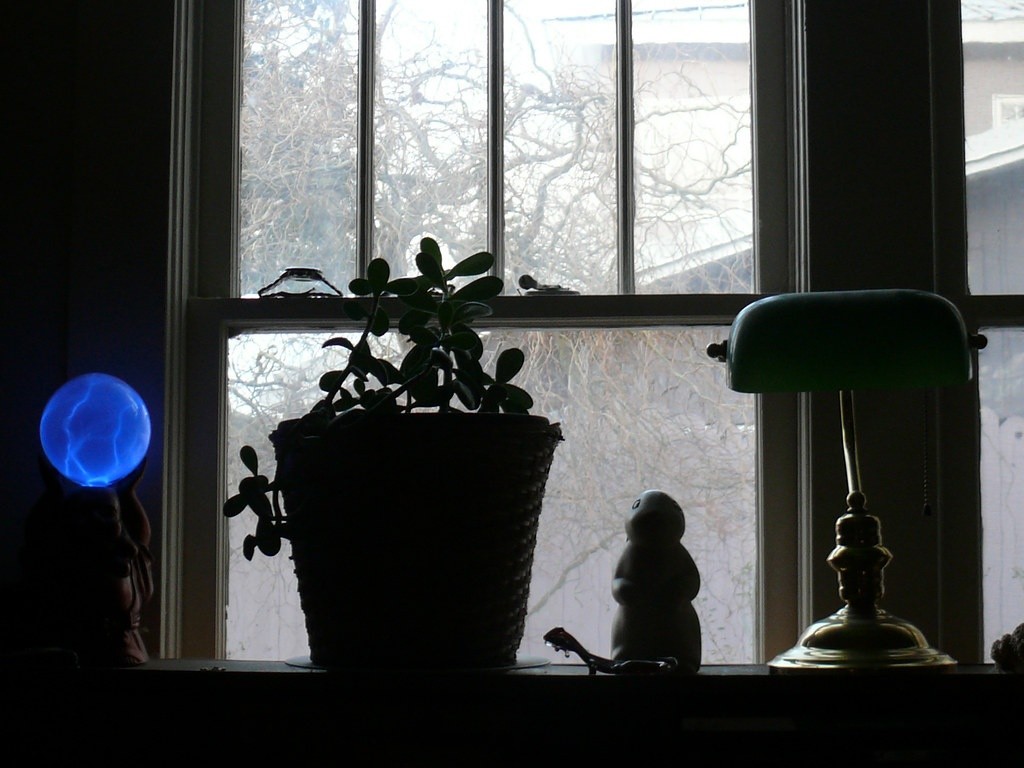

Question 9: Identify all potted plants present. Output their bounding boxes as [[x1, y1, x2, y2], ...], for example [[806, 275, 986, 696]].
[[219, 237, 564, 670]]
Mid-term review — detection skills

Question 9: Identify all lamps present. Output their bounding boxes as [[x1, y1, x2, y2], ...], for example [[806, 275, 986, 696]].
[[704, 288, 991, 671]]
